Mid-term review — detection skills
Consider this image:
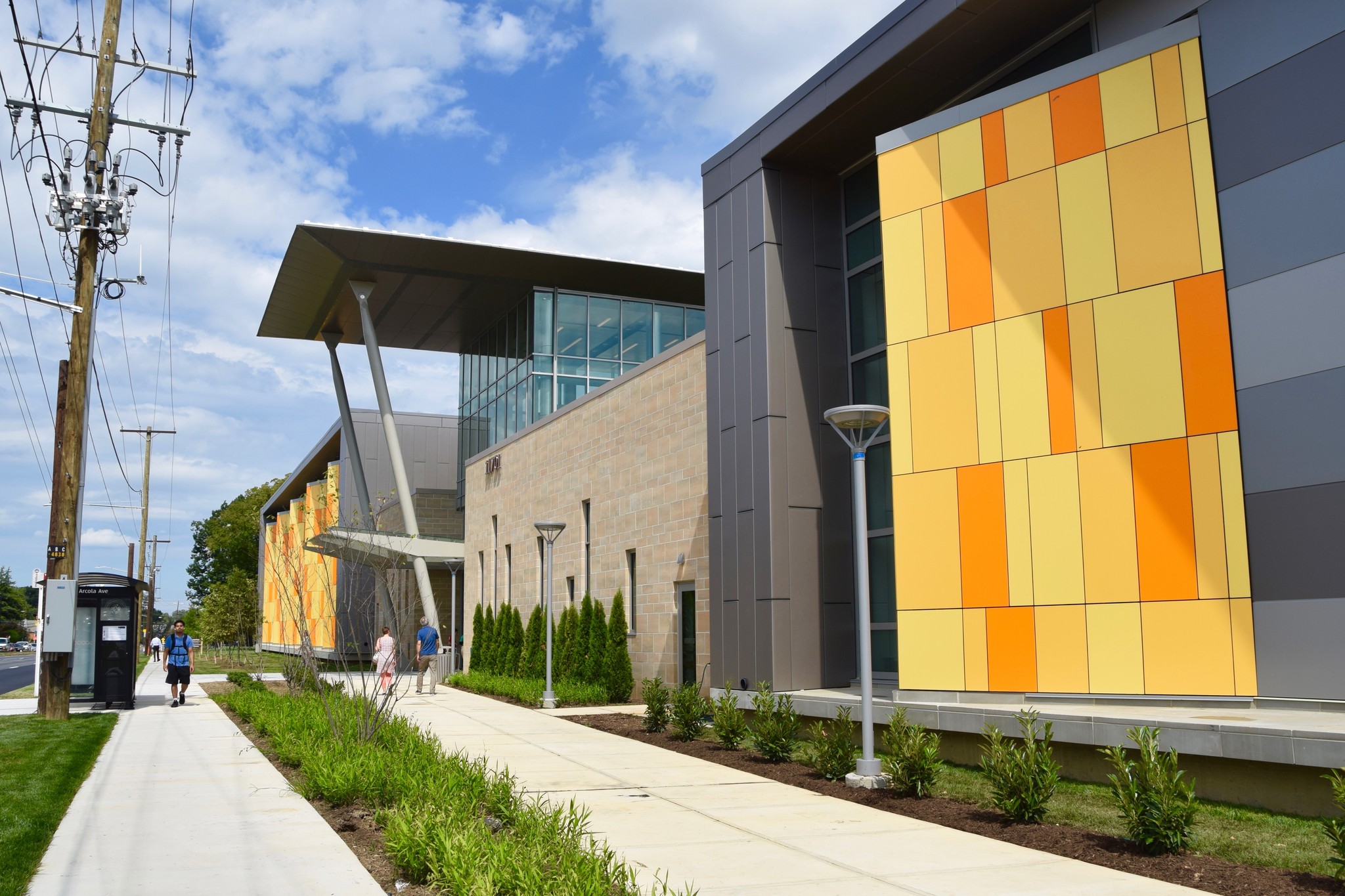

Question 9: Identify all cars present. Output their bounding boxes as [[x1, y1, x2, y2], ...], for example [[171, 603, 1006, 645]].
[[10, 643, 15, 651], [13, 643, 24, 652]]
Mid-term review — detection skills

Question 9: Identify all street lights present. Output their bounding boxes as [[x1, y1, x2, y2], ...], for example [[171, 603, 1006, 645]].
[[823, 403, 896, 790], [534, 522, 560, 709], [443, 559, 464, 676], [39, 497, 152, 665], [93, 565, 153, 658]]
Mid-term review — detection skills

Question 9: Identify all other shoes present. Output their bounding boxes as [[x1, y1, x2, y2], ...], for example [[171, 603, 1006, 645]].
[[153, 661, 156, 662], [389, 685, 392, 695], [430, 693, 436, 695], [415, 690, 421, 694], [179, 691, 185, 704], [381, 693, 386, 696], [157, 659, 160, 661], [171, 700, 178, 707]]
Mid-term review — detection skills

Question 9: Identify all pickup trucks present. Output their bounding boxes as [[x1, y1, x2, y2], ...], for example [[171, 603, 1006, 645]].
[[16, 642, 31, 652]]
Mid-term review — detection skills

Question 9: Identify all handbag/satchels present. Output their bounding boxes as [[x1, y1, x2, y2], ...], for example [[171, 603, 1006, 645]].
[[372, 650, 380, 665]]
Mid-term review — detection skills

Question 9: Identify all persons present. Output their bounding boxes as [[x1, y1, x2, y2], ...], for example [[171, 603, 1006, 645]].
[[375, 627, 396, 695], [415, 616, 440, 696], [163, 620, 195, 708], [150, 634, 162, 662], [449, 629, 463, 671]]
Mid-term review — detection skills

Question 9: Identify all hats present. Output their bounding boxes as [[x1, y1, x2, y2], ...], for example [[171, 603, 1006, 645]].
[[156, 634, 159, 636]]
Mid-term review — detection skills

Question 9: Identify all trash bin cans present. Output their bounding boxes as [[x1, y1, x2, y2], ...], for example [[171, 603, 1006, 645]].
[[436, 645, 457, 683]]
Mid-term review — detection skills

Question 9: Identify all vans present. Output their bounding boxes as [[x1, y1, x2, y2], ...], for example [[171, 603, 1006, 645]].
[[0, 638, 10, 652], [29, 643, 37, 652]]
